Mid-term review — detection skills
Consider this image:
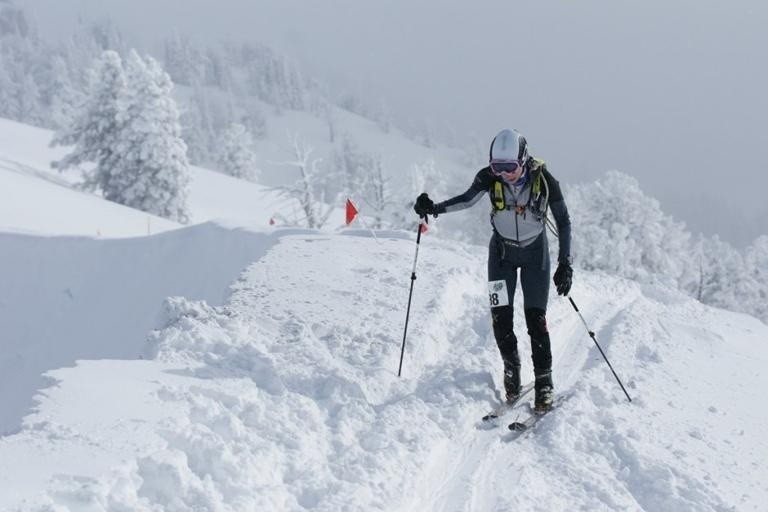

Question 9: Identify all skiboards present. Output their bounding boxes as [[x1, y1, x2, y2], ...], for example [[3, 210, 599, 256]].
[[482, 380, 573, 431]]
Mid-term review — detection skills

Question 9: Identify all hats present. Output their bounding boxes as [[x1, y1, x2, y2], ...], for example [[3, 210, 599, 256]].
[[488, 127, 528, 165]]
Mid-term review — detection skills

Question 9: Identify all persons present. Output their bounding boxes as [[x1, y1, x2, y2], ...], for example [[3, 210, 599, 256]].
[[417, 129, 572, 413]]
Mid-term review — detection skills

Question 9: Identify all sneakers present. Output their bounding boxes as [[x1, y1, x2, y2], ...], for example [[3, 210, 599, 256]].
[[502, 368, 523, 393], [535, 378, 554, 409]]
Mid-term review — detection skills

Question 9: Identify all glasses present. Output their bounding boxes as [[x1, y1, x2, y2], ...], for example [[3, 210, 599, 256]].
[[487, 159, 523, 177]]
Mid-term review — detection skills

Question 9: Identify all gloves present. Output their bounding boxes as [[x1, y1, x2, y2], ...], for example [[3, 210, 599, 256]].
[[553, 265, 574, 296], [414, 193, 435, 219]]
